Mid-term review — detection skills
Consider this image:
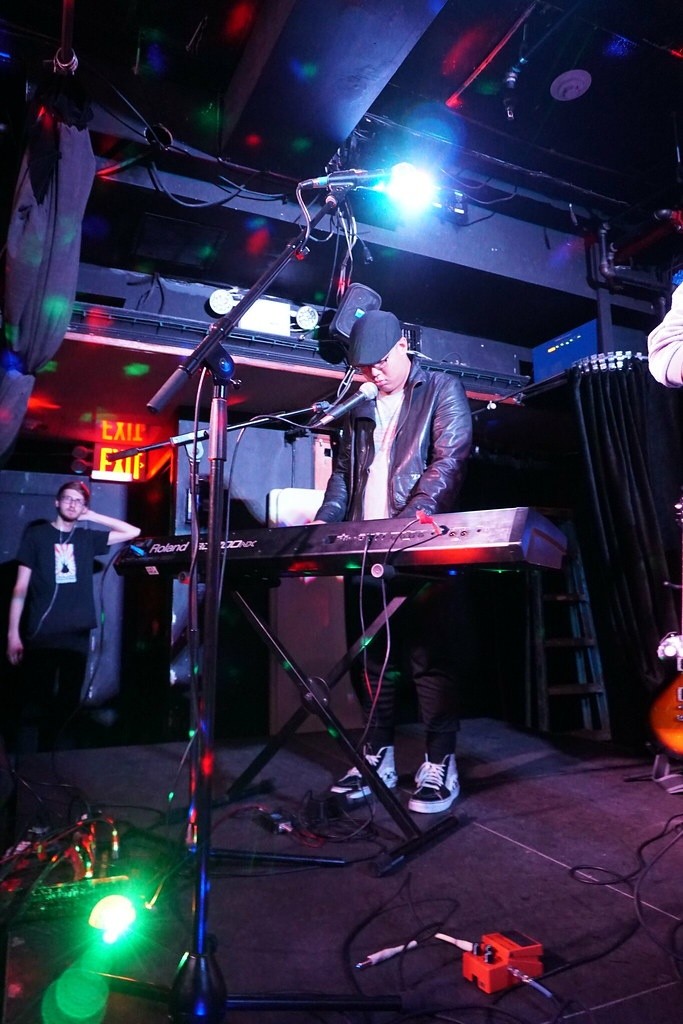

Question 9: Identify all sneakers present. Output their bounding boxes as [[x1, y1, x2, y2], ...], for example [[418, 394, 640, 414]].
[[408, 753, 462, 815], [331, 745, 397, 799]]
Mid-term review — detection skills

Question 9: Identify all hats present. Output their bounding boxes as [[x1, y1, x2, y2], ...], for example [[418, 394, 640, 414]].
[[348, 310, 402, 368]]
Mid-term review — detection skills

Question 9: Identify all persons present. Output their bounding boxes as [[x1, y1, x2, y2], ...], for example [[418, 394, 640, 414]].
[[305, 309, 474, 814], [648, 278, 683, 387], [7, 481, 140, 756]]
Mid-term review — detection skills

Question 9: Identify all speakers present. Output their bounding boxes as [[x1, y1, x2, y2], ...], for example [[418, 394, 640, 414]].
[[329, 282, 382, 346], [267, 575, 367, 736]]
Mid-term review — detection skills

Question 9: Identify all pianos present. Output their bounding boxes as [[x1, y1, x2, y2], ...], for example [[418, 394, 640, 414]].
[[114, 506, 570, 581]]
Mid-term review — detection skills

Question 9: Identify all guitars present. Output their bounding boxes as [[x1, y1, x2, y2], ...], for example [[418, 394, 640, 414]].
[[643, 668, 683, 763]]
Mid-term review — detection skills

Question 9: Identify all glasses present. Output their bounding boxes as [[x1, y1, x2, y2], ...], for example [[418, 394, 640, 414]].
[[354, 355, 393, 371], [58, 496, 86, 507]]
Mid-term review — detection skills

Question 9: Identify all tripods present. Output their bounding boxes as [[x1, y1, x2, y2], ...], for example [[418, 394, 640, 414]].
[[72, 185, 431, 1024]]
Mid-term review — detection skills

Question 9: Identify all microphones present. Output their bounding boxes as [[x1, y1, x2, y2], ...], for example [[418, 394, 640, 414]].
[[298, 163, 417, 190], [313, 382, 378, 429]]
